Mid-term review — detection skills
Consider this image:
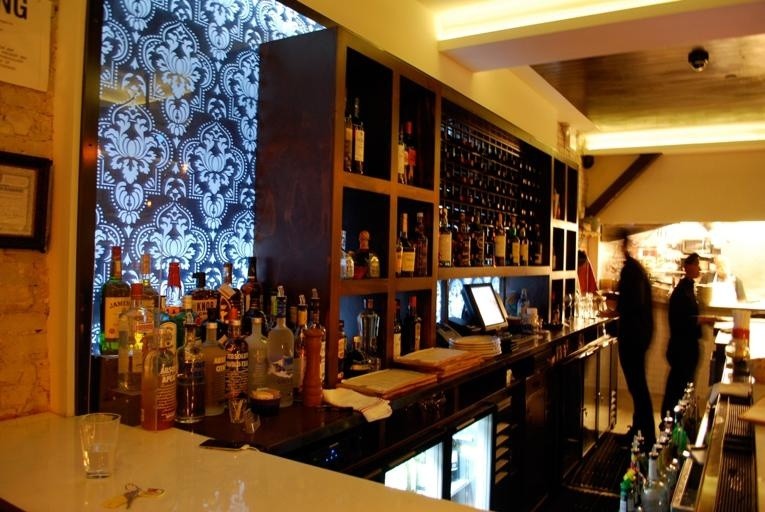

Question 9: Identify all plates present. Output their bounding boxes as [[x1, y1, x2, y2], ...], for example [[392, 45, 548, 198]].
[[448, 335, 504, 362]]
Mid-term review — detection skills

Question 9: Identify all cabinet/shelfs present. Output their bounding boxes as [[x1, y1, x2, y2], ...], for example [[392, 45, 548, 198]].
[[547, 149, 580, 324], [438, 80, 554, 326], [253, 25, 438, 389], [611, 338, 617, 431], [582, 349, 597, 462], [597, 341, 610, 441], [526, 349, 584, 512]]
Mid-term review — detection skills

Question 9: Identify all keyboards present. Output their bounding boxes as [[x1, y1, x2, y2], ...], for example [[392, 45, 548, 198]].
[[502, 334, 539, 346]]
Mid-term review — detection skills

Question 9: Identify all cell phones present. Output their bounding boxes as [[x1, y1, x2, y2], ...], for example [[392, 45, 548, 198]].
[[199, 439, 245, 450]]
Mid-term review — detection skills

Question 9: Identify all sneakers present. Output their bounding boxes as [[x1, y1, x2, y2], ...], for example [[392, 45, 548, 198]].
[[614, 430, 634, 446]]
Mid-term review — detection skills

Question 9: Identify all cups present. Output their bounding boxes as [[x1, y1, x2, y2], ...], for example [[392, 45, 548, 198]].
[[573, 294, 608, 320], [78, 411, 123, 482]]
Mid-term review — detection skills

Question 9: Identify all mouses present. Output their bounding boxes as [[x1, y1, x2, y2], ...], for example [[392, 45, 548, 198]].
[[519, 328, 532, 335]]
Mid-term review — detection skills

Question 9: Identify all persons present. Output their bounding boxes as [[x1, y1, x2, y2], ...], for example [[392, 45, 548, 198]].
[[605, 226, 657, 454], [659, 251, 720, 434]]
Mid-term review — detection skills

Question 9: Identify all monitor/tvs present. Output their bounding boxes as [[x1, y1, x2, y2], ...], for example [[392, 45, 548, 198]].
[[461, 283, 512, 339]]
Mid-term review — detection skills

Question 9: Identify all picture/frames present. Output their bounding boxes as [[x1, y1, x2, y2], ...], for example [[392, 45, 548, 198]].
[[1, 147, 53, 253]]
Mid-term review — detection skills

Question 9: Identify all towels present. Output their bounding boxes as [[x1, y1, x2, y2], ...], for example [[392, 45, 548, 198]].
[[341, 344, 484, 401]]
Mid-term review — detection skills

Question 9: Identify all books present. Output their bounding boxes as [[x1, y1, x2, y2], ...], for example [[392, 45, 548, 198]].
[[340, 347, 487, 401]]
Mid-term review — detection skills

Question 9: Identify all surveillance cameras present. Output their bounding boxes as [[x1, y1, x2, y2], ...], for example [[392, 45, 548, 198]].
[[688, 48, 709, 72]]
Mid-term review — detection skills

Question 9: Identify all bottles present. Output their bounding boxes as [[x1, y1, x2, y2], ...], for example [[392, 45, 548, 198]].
[[400, 107, 418, 184], [439, 124, 543, 267], [345, 94, 366, 174], [553, 303, 562, 323], [517, 288, 530, 318], [553, 187, 563, 219], [341, 207, 428, 278], [338, 293, 423, 380], [619, 382, 701, 512], [100, 247, 328, 431]]
